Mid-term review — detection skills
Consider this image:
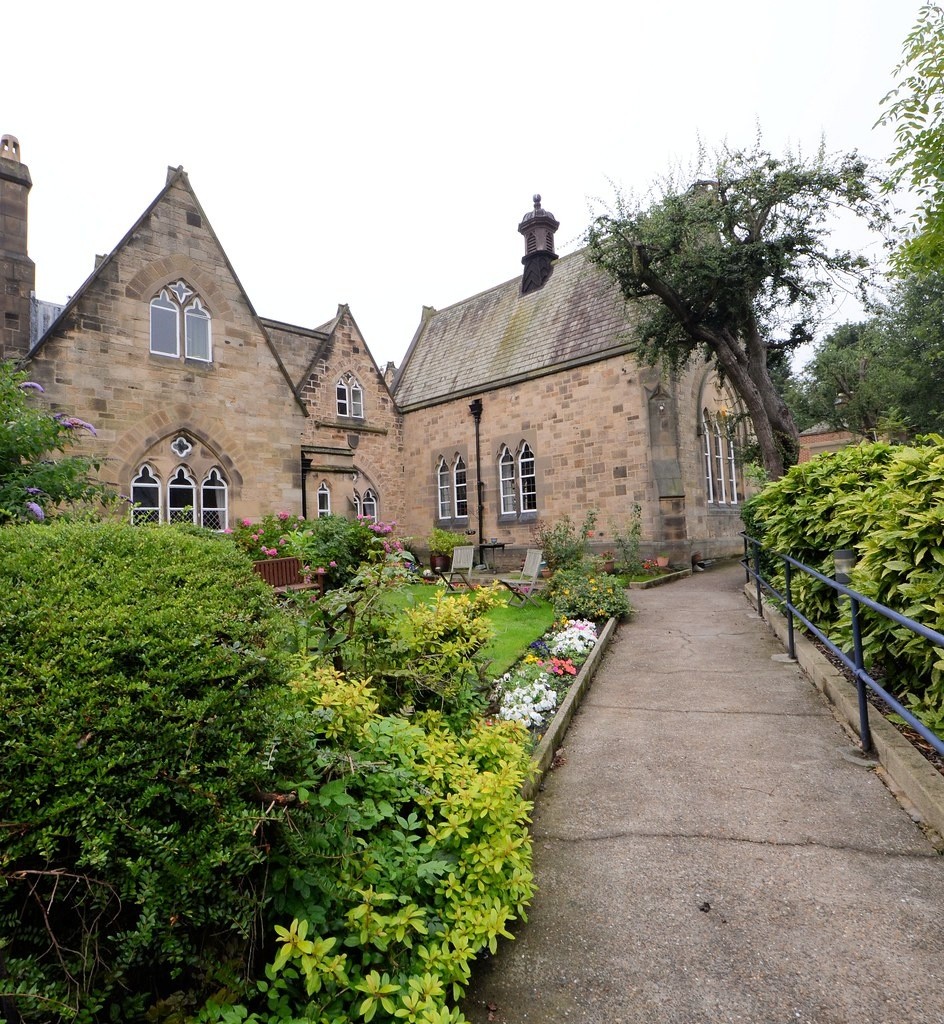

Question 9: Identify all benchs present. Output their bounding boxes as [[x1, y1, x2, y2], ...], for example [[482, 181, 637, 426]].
[[252, 556, 328, 598]]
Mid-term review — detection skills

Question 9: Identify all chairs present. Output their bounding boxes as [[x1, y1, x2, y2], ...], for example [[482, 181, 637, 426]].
[[439, 545, 476, 595], [498, 548, 544, 609]]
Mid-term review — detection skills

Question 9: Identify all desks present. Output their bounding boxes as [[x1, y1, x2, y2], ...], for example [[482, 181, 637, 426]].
[[479, 543, 505, 569]]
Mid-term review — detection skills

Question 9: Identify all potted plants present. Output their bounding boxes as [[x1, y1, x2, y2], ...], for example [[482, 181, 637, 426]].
[[656, 551, 671, 567], [424, 527, 474, 576]]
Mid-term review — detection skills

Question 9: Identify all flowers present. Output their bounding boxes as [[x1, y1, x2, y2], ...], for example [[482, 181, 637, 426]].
[[592, 551, 615, 561]]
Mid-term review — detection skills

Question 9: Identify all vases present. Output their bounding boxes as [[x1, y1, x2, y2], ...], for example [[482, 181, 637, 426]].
[[594, 560, 615, 575], [540, 569, 553, 578]]
